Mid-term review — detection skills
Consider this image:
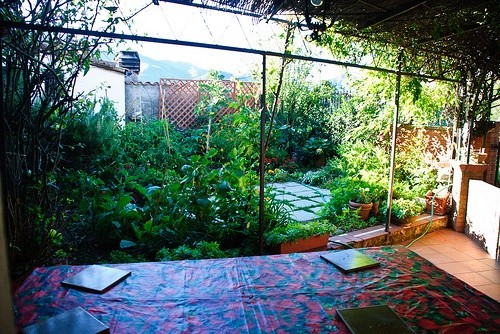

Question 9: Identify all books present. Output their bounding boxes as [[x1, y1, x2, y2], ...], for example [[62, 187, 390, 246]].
[[59, 264, 132, 294], [320, 249, 381, 274], [336, 304, 417, 334], [17, 306, 110, 334]]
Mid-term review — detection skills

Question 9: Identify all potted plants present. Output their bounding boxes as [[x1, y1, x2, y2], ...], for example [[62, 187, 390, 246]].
[[425, 189, 450, 215], [346, 173, 389, 221]]
